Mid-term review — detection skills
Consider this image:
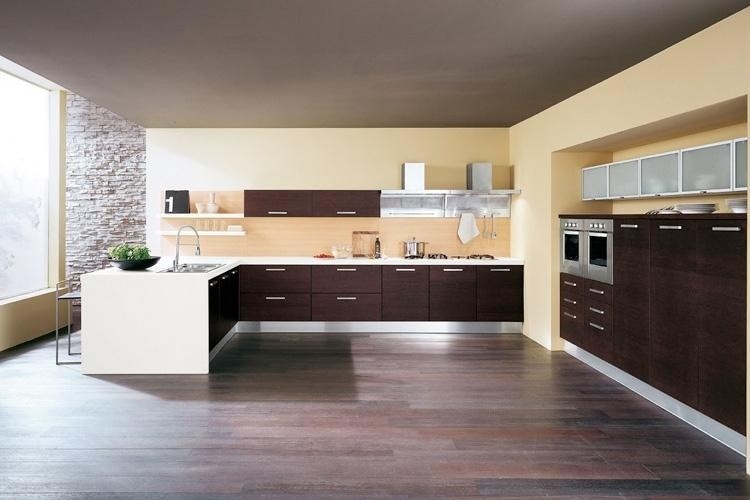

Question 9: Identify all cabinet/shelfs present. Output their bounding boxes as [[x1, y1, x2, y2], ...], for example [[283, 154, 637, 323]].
[[582, 165, 608, 201], [209, 266, 239, 363], [612, 219, 649, 384], [428, 265, 475, 322], [680, 139, 732, 197], [243, 189, 381, 218], [734, 136, 747, 193], [639, 152, 679, 197], [650, 219, 698, 412], [477, 265, 524, 321], [558, 272, 584, 351], [312, 264, 381, 321], [382, 266, 429, 321], [584, 279, 612, 363], [160, 213, 247, 236], [608, 159, 639, 200], [698, 219, 747, 436], [238, 265, 313, 320]]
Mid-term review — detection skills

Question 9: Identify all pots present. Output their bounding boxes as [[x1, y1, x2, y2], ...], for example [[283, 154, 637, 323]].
[[402, 237, 428, 258]]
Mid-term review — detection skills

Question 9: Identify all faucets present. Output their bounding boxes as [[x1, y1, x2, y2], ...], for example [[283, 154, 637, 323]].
[[172, 225, 201, 272]]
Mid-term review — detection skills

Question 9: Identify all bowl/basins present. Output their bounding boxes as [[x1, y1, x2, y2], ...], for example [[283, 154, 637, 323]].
[[227, 225, 243, 231], [107, 256, 161, 271], [330, 245, 354, 259]]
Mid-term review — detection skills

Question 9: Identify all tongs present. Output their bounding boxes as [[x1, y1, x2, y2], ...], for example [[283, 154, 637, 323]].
[[490, 214, 498, 239]]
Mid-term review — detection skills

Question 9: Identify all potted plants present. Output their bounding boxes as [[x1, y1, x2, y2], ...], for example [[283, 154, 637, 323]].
[[105, 243, 161, 270]]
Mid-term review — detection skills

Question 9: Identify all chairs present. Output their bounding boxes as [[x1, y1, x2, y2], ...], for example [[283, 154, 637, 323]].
[[56, 278, 81, 365]]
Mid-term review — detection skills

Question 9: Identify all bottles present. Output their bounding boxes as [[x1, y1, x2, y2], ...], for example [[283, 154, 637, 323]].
[[374, 237, 380, 259]]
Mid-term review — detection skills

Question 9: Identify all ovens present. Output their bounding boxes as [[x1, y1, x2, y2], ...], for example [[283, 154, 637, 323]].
[[557, 218, 614, 285]]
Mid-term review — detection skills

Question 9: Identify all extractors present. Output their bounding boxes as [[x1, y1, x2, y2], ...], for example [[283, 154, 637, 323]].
[[381, 162, 520, 217]]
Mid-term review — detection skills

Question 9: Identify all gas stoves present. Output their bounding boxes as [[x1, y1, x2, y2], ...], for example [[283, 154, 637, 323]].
[[427, 252, 494, 260]]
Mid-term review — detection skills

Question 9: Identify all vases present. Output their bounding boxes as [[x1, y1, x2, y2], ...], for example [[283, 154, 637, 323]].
[[196, 202, 206, 214]]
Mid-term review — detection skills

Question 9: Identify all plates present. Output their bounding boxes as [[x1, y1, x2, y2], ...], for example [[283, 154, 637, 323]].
[[725, 198, 747, 214], [656, 209, 680, 215], [676, 202, 717, 214]]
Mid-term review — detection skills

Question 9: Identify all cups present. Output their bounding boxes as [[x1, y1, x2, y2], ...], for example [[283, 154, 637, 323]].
[[195, 201, 206, 214], [191, 219, 226, 231]]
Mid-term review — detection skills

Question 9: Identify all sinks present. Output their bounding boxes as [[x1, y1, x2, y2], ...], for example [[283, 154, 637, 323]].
[[157, 263, 226, 273]]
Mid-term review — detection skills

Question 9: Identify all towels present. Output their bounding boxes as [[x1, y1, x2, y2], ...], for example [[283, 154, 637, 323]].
[[457, 212, 481, 245]]
